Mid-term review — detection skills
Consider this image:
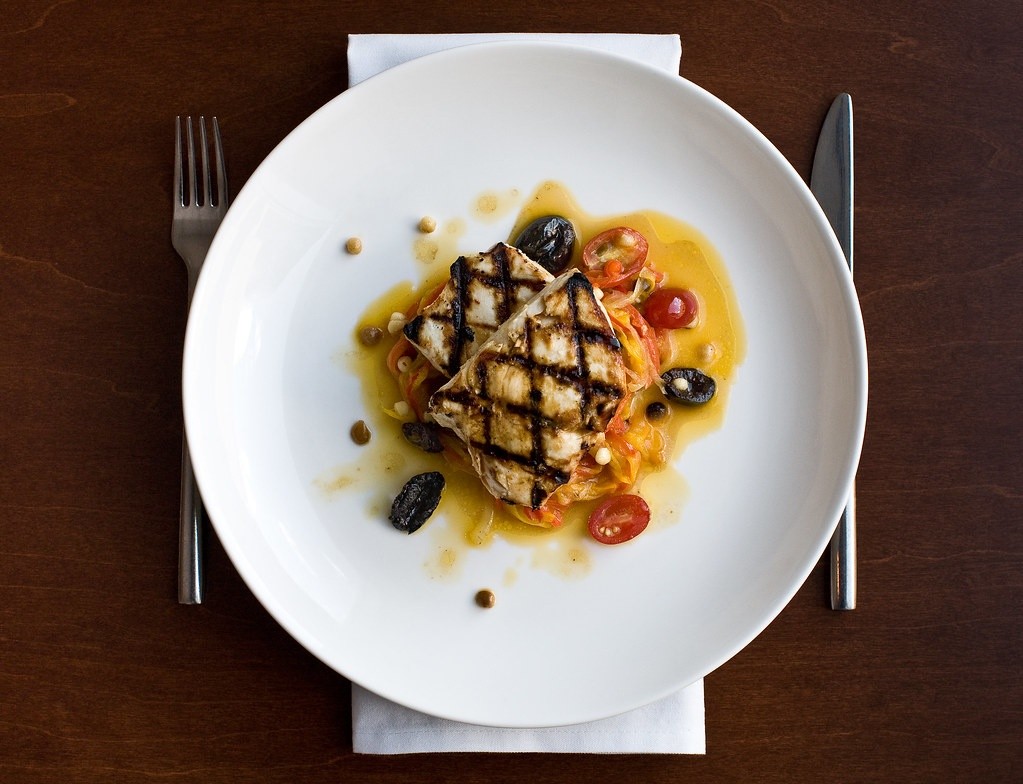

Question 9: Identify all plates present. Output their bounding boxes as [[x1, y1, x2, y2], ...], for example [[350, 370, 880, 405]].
[[181, 39, 870, 728]]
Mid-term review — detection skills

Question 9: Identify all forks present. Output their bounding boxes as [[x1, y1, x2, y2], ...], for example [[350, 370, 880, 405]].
[[170, 115, 225, 603]]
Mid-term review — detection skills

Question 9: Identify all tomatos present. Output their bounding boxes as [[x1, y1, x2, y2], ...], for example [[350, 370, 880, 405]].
[[580, 227, 649, 286], [588, 494, 650, 544], [644, 287, 697, 328], [625, 306, 660, 388]]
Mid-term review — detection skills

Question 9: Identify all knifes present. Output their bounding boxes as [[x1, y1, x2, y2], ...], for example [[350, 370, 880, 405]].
[[811, 93, 858, 609]]
